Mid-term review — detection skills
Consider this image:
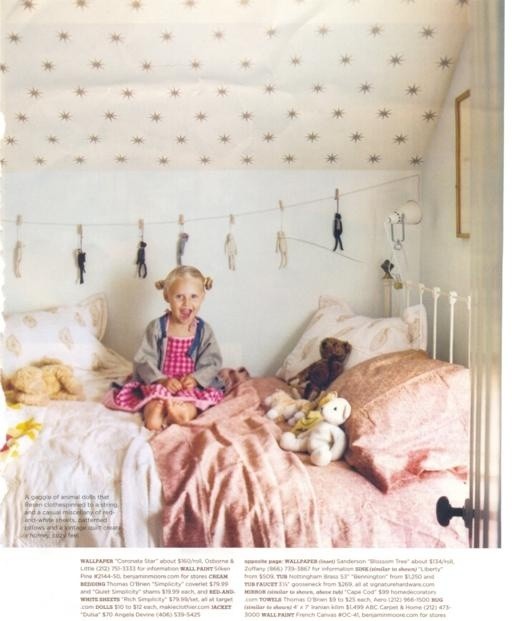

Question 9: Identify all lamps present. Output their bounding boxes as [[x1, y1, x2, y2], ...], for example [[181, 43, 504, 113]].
[[383, 200, 425, 291]]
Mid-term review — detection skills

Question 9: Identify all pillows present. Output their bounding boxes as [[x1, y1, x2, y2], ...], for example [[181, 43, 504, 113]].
[[276, 293, 428, 399], [316, 348, 475, 495], [1, 296, 137, 402]]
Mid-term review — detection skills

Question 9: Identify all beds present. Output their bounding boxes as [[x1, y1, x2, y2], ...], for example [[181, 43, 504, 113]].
[[0, 279, 500, 550]]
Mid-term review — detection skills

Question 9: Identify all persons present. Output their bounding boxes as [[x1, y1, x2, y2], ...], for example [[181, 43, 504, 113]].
[[103, 266, 225, 431]]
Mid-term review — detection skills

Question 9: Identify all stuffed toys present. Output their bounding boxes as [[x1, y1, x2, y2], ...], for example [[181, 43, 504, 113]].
[[176, 232, 189, 266], [262, 389, 310, 427], [77, 249, 86, 285], [278, 397, 352, 466], [290, 337, 352, 403], [137, 242, 148, 278], [225, 234, 236, 270], [333, 213, 344, 252], [0, 359, 87, 405], [14, 241, 23, 278], [276, 230, 288, 268]]
